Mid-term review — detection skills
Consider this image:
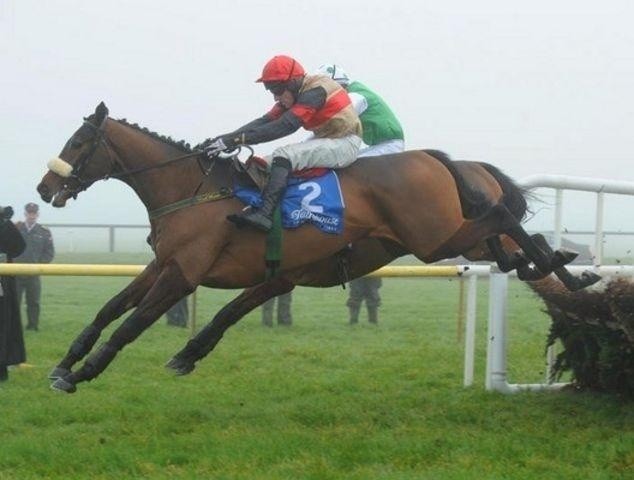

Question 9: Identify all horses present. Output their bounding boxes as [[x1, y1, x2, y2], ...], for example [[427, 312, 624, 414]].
[[146, 159, 603, 377], [36, 99, 582, 394]]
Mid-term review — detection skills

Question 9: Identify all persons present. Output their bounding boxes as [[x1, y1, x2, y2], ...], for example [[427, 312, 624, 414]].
[[0, 205, 28, 381], [262, 286, 293, 329], [206, 52, 364, 233], [14, 202, 55, 329], [318, 62, 406, 158], [348, 276, 383, 327]]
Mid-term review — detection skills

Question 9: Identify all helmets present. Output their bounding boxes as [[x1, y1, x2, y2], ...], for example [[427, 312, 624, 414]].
[[313, 64, 350, 86], [255, 56, 305, 90]]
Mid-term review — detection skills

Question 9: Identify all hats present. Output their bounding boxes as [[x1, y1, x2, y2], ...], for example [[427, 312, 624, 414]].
[[25, 203, 39, 213]]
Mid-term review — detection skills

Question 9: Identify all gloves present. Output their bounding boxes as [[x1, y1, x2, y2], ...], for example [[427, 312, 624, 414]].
[[202, 133, 245, 160]]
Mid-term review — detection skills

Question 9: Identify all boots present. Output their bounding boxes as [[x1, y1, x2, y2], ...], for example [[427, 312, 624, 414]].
[[227, 157, 291, 232]]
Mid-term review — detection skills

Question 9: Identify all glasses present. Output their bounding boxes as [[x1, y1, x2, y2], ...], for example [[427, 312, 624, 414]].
[[269, 80, 286, 95]]
